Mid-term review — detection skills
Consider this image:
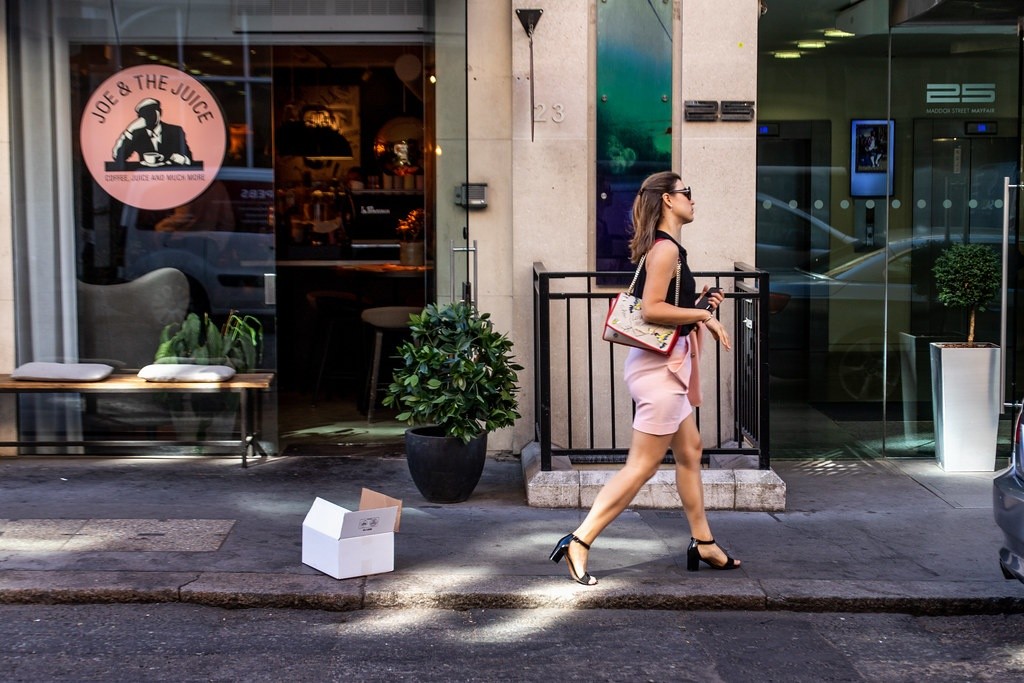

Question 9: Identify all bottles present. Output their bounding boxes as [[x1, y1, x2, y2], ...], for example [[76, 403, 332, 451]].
[[381, 143, 424, 191]]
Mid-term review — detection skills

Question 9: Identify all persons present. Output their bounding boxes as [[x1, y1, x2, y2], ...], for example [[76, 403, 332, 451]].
[[231, 160, 396, 242], [550, 171, 741, 585], [154, 180, 236, 262]]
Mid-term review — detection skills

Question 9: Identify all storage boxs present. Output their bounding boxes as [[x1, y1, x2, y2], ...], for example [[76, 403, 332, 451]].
[[300, 489, 402, 581]]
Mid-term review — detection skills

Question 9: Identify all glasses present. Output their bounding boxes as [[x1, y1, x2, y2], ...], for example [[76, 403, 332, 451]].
[[664, 185, 691, 201]]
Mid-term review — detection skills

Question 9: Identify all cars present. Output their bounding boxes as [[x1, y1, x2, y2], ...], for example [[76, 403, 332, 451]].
[[762, 235, 1024, 410]]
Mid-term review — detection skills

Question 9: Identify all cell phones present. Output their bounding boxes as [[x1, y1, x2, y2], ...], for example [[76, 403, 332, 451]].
[[697, 286, 724, 310]]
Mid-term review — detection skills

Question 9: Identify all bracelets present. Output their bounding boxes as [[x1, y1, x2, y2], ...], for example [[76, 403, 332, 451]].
[[702, 314, 713, 323]]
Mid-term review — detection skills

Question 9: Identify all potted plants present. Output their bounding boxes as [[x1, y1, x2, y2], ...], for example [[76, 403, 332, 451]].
[[377, 297, 525, 505], [396, 208, 424, 265], [898, 235, 964, 446], [927, 238, 1002, 473], [153, 309, 264, 454]]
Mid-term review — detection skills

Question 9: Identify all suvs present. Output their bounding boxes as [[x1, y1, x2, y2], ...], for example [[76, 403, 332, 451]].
[[598, 184, 864, 286]]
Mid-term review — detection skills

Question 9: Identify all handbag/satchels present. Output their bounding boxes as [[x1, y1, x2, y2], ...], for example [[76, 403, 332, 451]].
[[603, 238, 681, 357]]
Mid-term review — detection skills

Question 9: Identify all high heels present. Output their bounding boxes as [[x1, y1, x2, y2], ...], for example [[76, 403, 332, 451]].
[[687, 537, 741, 572], [549, 533, 597, 586]]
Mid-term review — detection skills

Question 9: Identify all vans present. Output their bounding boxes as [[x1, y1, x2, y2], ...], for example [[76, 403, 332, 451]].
[[115, 168, 273, 342]]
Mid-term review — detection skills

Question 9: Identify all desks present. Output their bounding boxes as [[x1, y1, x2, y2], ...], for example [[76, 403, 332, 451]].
[[0, 373, 274, 465]]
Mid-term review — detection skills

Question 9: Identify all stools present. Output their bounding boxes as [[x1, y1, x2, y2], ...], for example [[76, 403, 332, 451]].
[[362, 304, 424, 423]]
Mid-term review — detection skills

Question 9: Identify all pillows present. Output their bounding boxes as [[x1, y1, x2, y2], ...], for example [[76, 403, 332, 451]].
[[136, 364, 236, 382], [10, 361, 115, 382]]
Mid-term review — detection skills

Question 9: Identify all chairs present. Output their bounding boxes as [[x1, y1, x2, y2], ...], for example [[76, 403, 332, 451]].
[[76, 265, 197, 428]]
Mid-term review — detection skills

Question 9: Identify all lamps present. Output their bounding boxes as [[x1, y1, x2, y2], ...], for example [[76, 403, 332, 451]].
[[302, 103, 355, 160]]
